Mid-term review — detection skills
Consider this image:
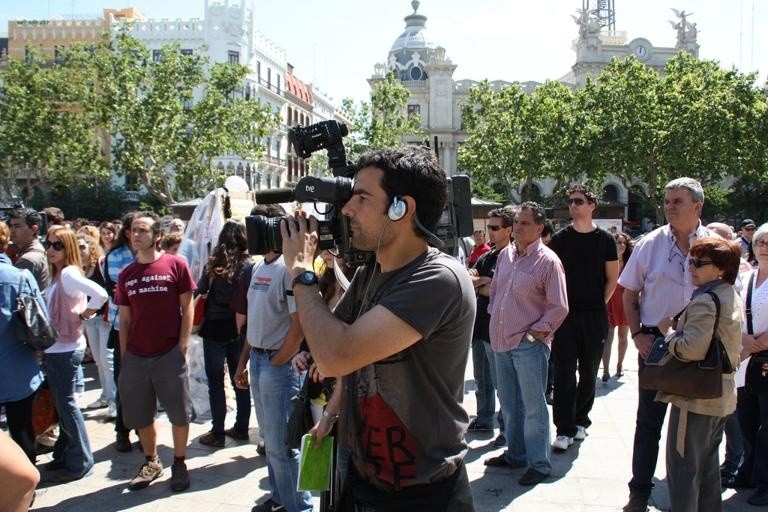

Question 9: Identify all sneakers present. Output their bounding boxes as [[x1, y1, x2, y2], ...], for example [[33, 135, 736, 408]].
[[43, 400, 294, 507]]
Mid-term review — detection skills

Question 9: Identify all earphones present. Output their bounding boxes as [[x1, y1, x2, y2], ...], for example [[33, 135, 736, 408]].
[[387, 196, 408, 221]]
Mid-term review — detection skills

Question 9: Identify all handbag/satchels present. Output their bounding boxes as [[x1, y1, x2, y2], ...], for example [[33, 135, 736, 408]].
[[11, 291, 56, 351], [192, 292, 209, 332], [107, 325, 119, 346], [641, 335, 733, 398], [745, 351, 768, 402], [286, 390, 315, 449], [32, 388, 59, 441]]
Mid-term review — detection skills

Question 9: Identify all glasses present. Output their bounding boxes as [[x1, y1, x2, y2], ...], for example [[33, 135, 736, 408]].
[[44, 240, 63, 250], [487, 225, 501, 231], [690, 259, 711, 268], [568, 198, 592, 206]]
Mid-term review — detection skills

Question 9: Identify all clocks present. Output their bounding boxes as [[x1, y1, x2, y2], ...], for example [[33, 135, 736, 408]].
[[635, 46, 646, 59]]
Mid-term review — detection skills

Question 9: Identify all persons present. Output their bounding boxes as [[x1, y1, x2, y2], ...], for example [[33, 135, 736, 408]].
[[279, 148, 476, 510], [0, 178, 768, 512]]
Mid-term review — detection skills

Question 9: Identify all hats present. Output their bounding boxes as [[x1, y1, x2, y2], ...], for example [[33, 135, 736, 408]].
[[741, 219, 755, 228]]
